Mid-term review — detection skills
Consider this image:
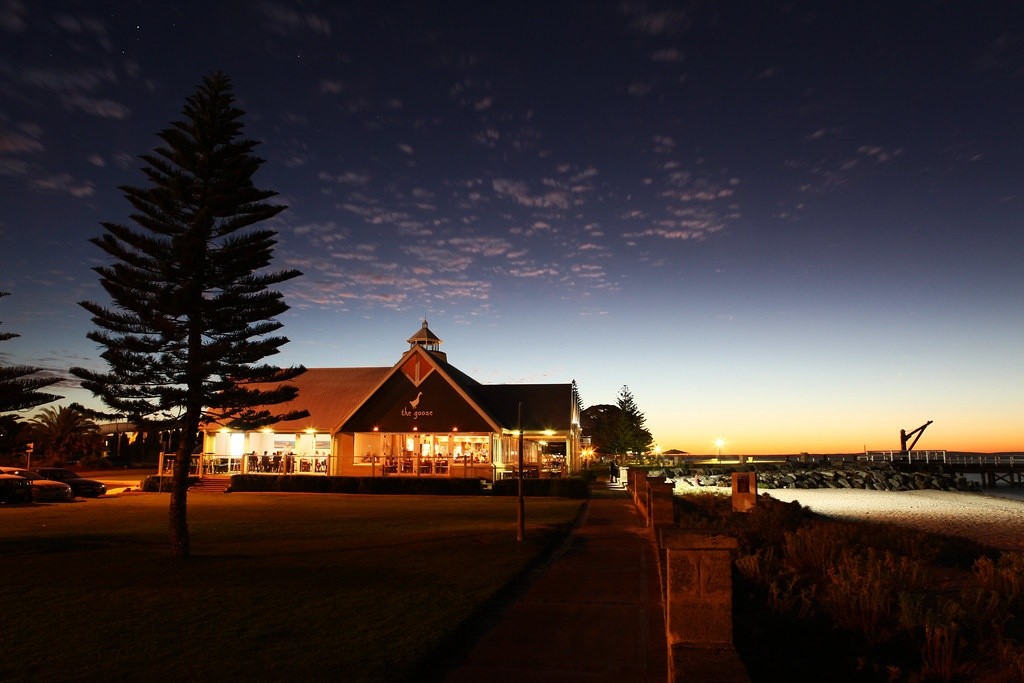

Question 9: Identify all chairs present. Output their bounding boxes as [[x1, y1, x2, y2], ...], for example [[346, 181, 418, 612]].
[[364, 452, 489, 475], [190, 456, 327, 475]]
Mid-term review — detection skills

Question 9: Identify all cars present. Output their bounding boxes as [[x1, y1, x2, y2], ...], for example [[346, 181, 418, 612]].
[[0, 469, 32, 503], [0, 466, 71, 500], [37, 467, 107, 498]]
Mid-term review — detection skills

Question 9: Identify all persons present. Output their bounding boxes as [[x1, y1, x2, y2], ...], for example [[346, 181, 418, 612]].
[[247, 451, 326, 473], [367, 450, 488, 477], [609, 458, 619, 484]]
